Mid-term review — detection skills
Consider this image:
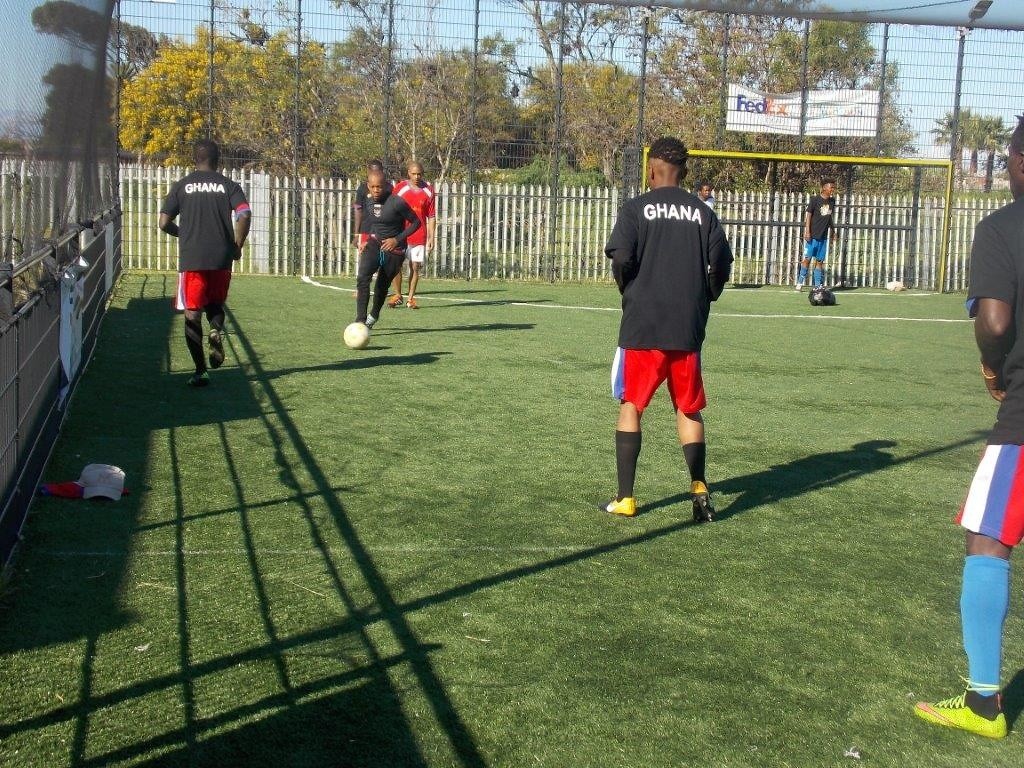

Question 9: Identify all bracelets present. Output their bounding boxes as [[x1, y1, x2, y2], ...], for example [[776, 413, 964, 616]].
[[980, 356, 999, 379]]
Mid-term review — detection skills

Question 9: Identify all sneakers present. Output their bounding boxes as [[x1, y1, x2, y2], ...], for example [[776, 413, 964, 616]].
[[406, 301, 419, 309], [185, 372, 211, 386], [386, 295, 403, 307], [598, 497, 637, 517], [690, 481, 716, 523], [207, 329, 226, 369], [914, 675, 1009, 739]]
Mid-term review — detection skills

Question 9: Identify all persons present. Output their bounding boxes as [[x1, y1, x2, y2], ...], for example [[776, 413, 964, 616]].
[[355, 160, 434, 334], [604, 140, 734, 523], [912, 130, 1024, 742], [158, 139, 253, 388], [795, 177, 838, 290]]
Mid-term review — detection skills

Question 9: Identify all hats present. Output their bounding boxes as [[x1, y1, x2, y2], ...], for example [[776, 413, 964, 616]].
[[80, 463, 126, 501]]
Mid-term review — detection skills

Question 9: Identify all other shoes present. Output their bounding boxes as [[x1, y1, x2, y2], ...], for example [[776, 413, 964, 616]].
[[364, 314, 376, 330], [796, 283, 803, 290]]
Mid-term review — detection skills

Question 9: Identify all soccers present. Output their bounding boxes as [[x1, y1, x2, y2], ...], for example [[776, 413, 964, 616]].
[[809, 290, 827, 306], [343, 322, 370, 348]]
